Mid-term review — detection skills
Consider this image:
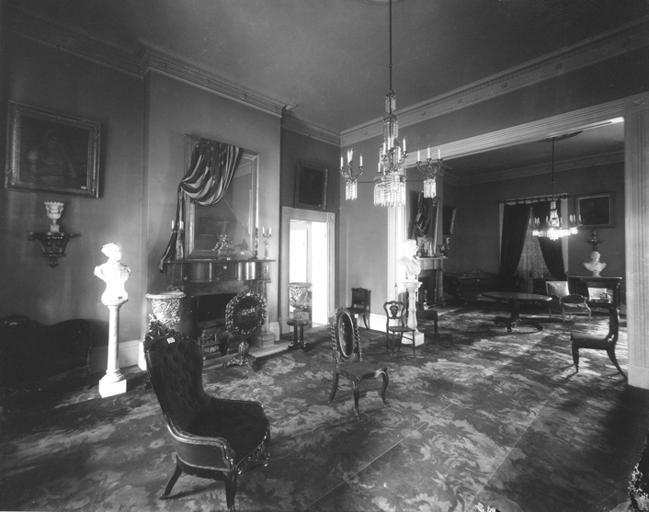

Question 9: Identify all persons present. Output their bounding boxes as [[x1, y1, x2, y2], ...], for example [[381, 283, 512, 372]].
[[94, 243, 131, 301], [584, 250, 607, 272], [399, 239, 421, 276]]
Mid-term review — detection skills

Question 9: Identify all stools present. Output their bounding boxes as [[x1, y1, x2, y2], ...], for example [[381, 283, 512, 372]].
[[286, 320, 311, 350]]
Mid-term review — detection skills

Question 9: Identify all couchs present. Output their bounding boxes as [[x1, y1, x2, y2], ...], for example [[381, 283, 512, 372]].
[[0, 316, 95, 409]]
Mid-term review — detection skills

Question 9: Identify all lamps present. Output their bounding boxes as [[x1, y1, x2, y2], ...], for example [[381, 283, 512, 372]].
[[339, 1, 443, 207], [528, 139, 583, 242]]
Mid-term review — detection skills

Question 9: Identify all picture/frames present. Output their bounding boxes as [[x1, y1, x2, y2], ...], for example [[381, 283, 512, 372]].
[[575, 191, 617, 229], [4, 98, 100, 200]]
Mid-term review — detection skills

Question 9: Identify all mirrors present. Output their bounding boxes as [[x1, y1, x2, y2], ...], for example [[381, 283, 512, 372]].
[[184, 134, 260, 258]]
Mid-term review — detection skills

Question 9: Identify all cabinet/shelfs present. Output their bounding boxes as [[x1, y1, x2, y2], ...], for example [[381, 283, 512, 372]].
[[415, 254, 447, 309], [166, 259, 277, 366]]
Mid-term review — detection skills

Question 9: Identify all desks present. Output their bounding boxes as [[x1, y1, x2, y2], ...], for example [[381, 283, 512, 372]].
[[568, 276, 623, 319], [483, 291, 552, 333]]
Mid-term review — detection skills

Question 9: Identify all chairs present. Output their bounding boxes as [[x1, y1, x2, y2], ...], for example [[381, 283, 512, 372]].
[[546, 280, 593, 322], [383, 301, 416, 358], [570, 306, 627, 378], [345, 288, 371, 331], [328, 308, 389, 415], [143, 312, 271, 511], [223, 291, 267, 367]]
